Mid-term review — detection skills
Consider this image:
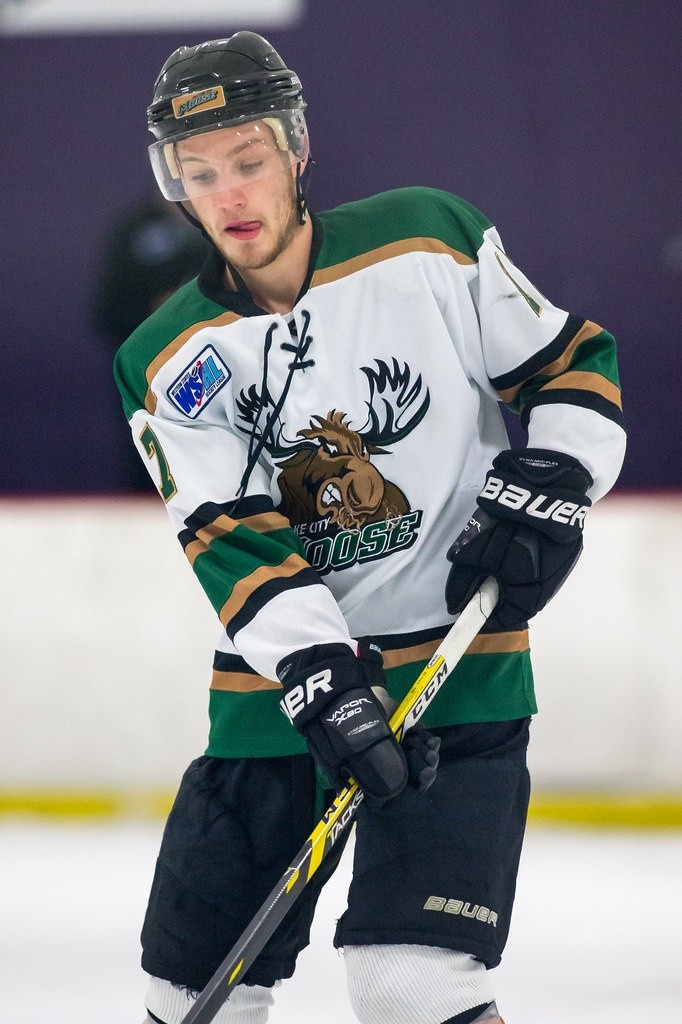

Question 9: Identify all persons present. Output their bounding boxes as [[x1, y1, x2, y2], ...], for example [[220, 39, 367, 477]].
[[113, 32, 626, 1024], [93, 181, 206, 495]]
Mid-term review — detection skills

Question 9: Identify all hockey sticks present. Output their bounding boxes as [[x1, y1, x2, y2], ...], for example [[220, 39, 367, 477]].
[[181, 579, 501, 1024]]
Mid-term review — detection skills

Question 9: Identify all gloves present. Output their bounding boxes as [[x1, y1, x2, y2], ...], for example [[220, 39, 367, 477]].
[[276, 643, 440, 813], [444, 449, 595, 630]]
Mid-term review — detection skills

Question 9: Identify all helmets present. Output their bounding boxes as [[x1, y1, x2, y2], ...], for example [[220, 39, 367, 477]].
[[146, 31, 309, 202]]
